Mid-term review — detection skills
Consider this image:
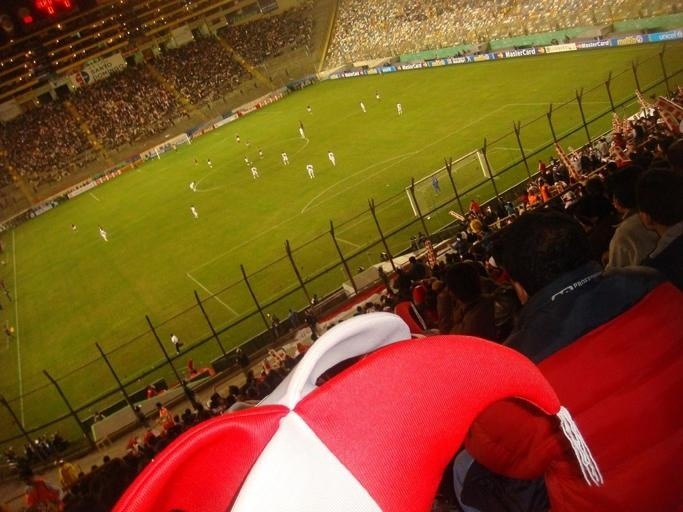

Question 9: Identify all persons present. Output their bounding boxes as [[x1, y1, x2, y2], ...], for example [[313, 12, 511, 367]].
[[1, 0, 683, 512]]
